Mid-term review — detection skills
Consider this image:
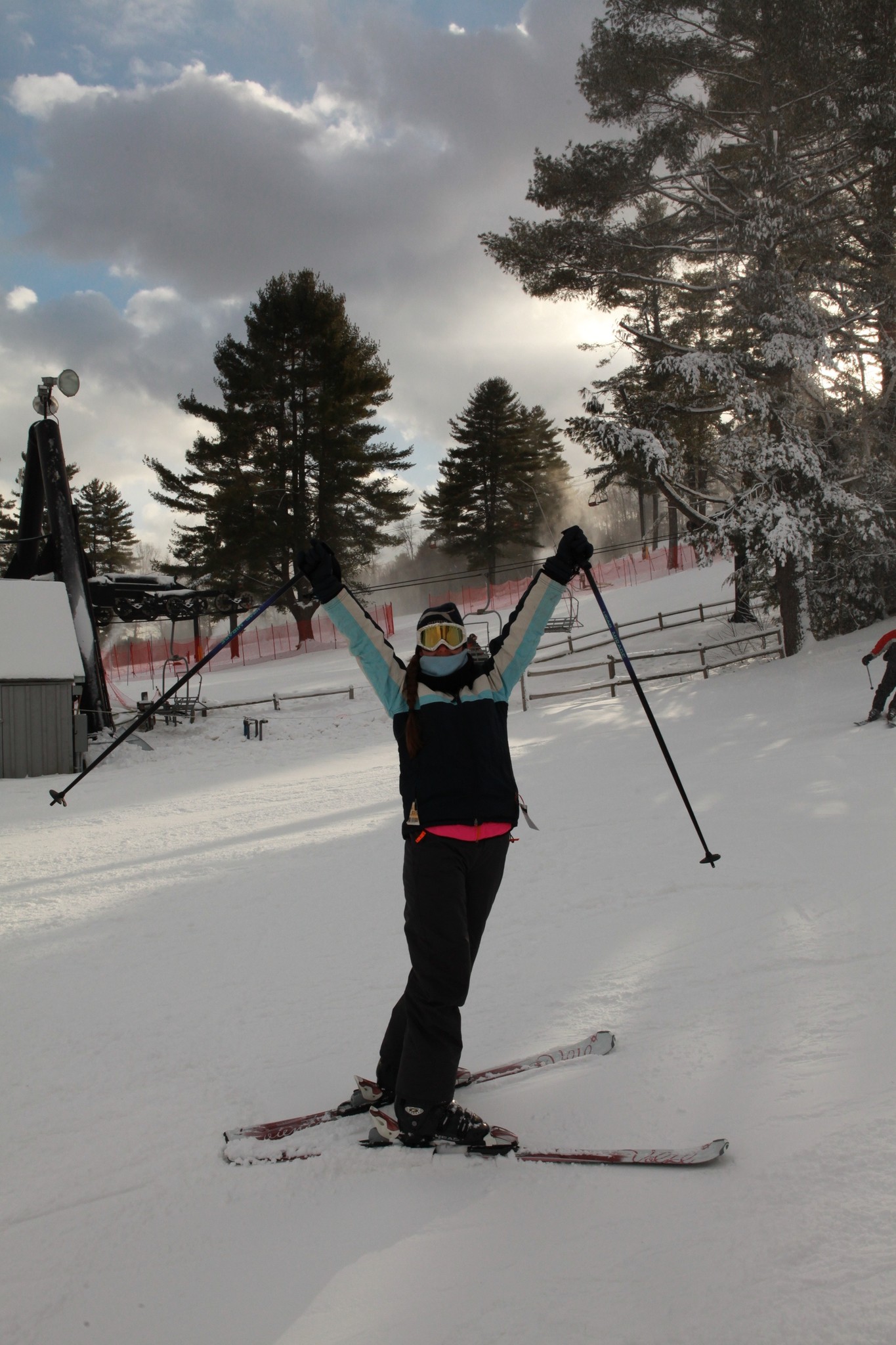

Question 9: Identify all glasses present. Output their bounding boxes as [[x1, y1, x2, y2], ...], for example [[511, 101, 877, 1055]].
[[416, 622, 466, 651]]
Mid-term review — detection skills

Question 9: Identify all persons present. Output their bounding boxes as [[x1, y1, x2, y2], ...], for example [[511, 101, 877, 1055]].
[[298, 525, 593, 1145], [467, 634, 489, 661], [861, 629, 896, 721]]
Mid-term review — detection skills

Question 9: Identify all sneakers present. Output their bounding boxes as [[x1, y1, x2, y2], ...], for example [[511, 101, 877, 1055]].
[[888, 708, 896, 720], [869, 708, 881, 720], [374, 1057, 398, 1088], [399, 1101, 489, 1141]]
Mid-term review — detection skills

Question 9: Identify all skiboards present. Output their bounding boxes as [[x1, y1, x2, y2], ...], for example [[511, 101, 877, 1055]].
[[853, 713, 896, 727], [223, 1029, 733, 1166]]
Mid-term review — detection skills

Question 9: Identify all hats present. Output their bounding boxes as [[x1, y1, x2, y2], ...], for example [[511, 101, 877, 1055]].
[[416, 602, 464, 630], [470, 633, 475, 638]]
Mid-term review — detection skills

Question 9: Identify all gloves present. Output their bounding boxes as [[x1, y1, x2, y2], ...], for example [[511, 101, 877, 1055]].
[[862, 654, 873, 665], [298, 538, 343, 603], [555, 525, 593, 569]]
